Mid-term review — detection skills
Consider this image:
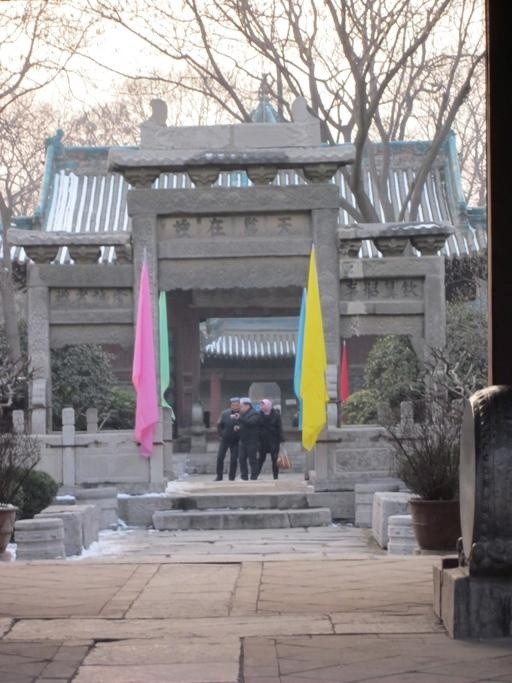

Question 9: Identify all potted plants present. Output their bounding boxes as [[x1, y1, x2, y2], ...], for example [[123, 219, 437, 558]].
[[378, 385, 460, 550], [0, 414, 42, 553]]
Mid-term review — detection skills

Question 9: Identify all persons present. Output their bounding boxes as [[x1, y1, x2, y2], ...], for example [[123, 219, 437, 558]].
[[256, 399, 285, 479], [215, 397, 240, 481], [230, 398, 259, 480]]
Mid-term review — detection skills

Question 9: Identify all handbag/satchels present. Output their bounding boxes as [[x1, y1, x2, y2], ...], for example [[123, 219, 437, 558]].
[[276, 454, 293, 469]]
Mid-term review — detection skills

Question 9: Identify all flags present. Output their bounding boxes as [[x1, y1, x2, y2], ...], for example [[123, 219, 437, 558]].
[[293, 285, 308, 430], [158, 291, 177, 421], [300, 248, 330, 451], [132, 258, 160, 458], [340, 342, 348, 402]]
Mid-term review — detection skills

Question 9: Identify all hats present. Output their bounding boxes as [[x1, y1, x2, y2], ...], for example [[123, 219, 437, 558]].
[[230, 397, 240, 402], [239, 397, 251, 403]]
